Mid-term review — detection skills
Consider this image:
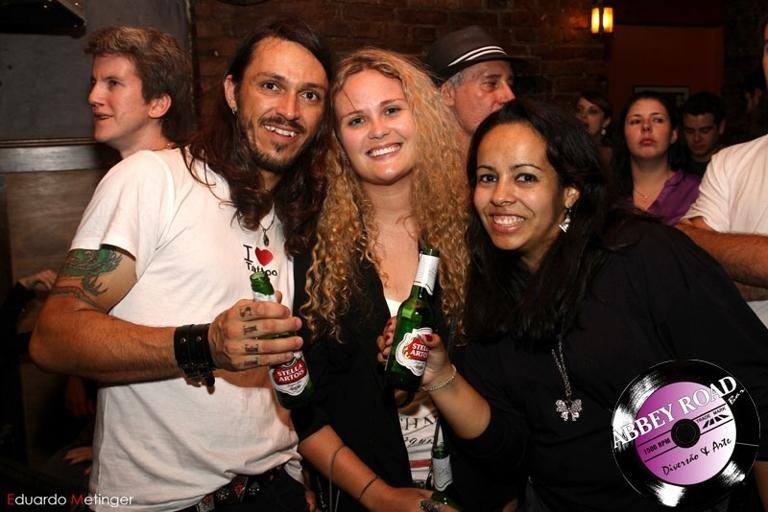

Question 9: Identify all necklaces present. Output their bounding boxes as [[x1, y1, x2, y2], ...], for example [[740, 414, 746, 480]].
[[538, 338, 585, 429], [634, 187, 655, 206], [257, 219, 277, 248]]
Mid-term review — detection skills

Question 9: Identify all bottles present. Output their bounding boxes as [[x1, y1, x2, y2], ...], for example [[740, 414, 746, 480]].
[[249, 270, 316, 411], [384, 246, 441, 394], [428, 446, 461, 512]]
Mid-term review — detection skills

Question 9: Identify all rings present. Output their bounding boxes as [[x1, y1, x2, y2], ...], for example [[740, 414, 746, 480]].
[[418, 497, 447, 511]]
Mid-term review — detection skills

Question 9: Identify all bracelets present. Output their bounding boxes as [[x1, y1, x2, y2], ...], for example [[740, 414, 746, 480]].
[[173, 322, 195, 393], [420, 365, 460, 393], [192, 324, 219, 396], [356, 475, 382, 506]]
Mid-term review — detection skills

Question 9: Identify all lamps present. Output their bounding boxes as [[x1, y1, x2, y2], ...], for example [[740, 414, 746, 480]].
[[589, 0, 616, 42]]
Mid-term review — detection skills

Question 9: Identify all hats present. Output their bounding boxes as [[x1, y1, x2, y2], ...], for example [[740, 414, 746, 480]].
[[428, 24, 530, 83]]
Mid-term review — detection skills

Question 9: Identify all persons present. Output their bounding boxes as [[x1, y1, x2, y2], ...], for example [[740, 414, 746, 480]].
[[375, 91, 768, 510], [613, 90, 699, 225], [64, 441, 94, 476], [663, 16, 768, 333], [276, 42, 478, 511], [82, 22, 187, 161], [732, 74, 768, 145], [24, 14, 341, 510], [671, 91, 739, 175], [570, 93, 629, 192], [1, 197, 60, 342], [431, 21, 537, 142]]
